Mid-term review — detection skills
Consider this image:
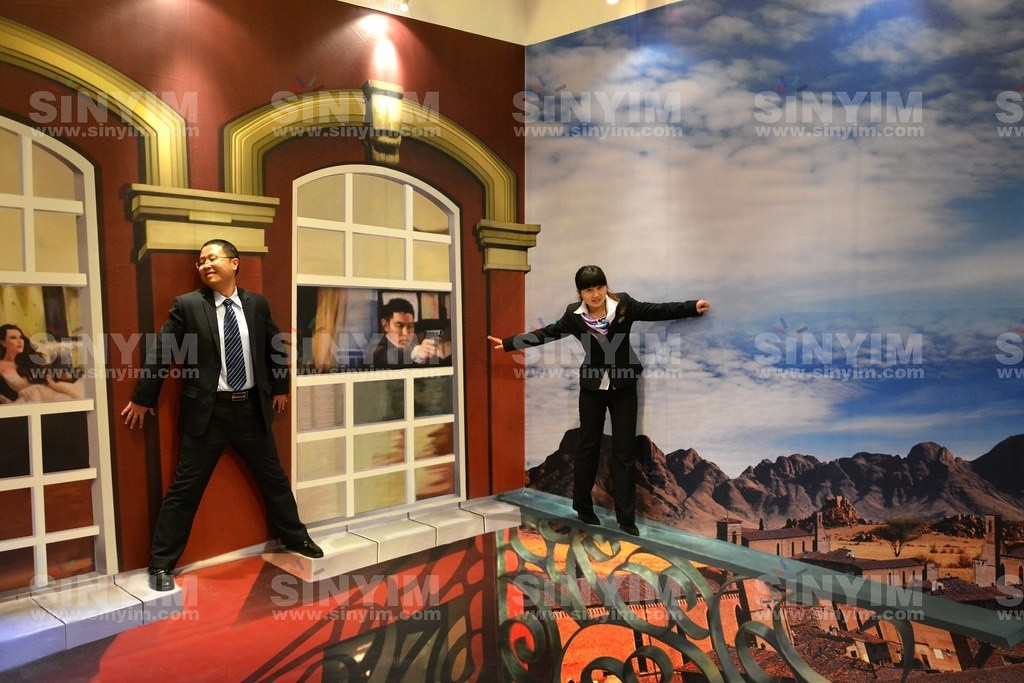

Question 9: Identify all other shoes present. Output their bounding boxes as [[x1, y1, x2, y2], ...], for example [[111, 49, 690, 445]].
[[578, 510, 601, 525], [620, 523, 640, 537]]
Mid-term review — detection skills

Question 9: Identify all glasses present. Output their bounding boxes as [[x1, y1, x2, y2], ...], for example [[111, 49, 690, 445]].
[[195, 255, 235, 269]]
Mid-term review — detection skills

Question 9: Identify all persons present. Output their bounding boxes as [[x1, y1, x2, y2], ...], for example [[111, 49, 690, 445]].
[[120, 238, 325, 592], [0, 324, 84, 404], [487, 264, 711, 536], [373, 298, 452, 370]]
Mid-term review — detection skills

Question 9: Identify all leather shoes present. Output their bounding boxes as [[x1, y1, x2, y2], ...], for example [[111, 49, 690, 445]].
[[286, 534, 324, 558], [148, 567, 175, 591]]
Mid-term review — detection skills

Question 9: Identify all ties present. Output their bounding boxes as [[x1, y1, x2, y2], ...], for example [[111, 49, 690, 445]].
[[223, 298, 248, 392]]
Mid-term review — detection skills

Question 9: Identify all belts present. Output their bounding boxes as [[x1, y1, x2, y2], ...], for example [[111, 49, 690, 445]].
[[215, 387, 257, 402]]
